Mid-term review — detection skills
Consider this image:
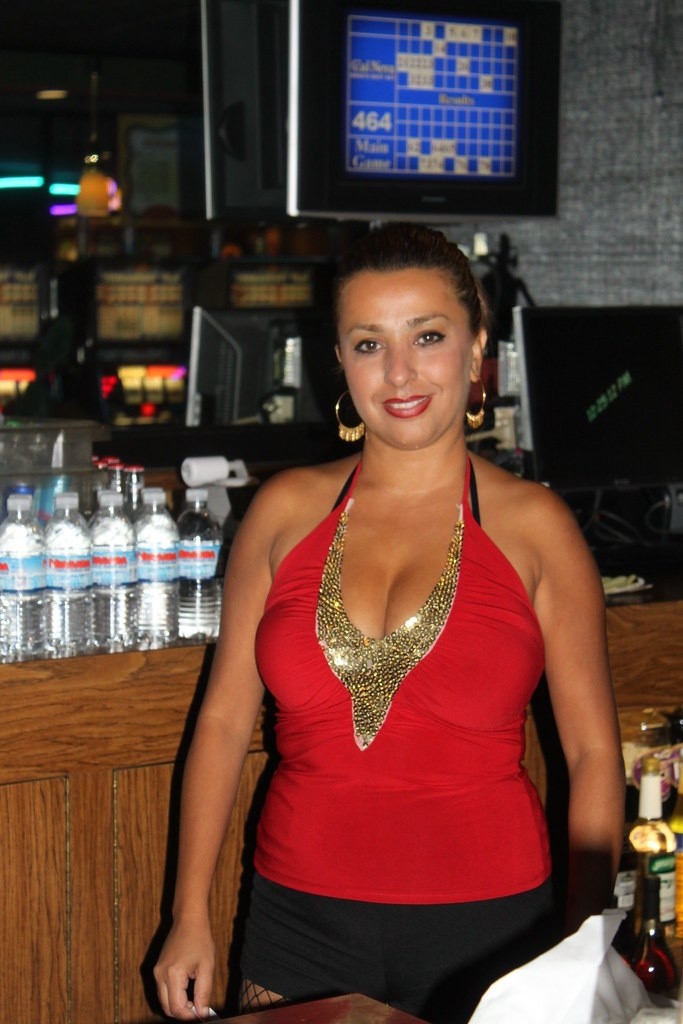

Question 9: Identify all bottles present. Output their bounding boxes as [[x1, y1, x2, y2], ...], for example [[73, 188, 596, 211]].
[[614, 706, 683, 995], [178, 488, 223, 639], [0, 455, 178, 665]]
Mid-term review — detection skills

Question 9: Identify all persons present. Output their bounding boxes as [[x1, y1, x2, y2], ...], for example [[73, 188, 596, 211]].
[[154, 221, 626, 1020]]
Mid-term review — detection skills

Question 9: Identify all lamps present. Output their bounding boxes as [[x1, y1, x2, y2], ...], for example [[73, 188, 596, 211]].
[[77, 69, 109, 218]]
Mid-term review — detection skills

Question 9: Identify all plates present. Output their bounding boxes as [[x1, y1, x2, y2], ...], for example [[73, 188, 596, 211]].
[[603, 576, 644, 595]]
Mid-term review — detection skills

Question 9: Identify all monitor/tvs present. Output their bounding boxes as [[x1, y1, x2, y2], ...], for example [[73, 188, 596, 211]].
[[183, 304, 347, 427], [514, 302, 682, 549], [278, 0, 563, 221]]
